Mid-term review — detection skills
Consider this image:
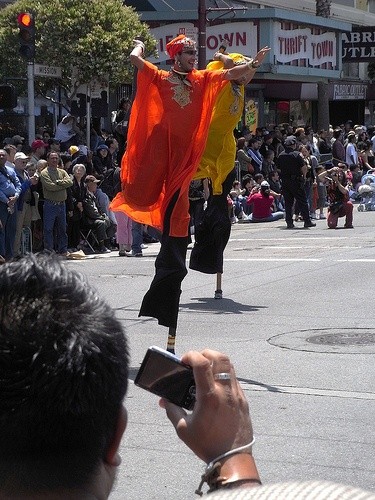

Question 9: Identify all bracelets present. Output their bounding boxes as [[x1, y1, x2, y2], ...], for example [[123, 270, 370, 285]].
[[136, 44, 144, 53], [247, 61, 258, 71], [219, 55, 223, 59], [225, 478, 261, 489]]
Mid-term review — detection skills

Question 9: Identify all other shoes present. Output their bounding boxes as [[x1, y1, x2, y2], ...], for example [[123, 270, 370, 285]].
[[304, 222, 317, 227], [105, 239, 118, 250], [320, 214, 326, 219], [287, 223, 294, 228], [99, 240, 111, 253], [120, 242, 149, 257]]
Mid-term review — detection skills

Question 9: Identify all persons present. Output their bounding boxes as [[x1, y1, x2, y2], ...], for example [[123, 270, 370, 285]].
[[0, 96, 375, 262], [108, 34, 272, 238], [191, 50, 255, 196], [0, 251, 263, 500]]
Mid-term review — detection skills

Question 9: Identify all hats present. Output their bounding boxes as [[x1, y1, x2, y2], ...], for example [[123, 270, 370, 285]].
[[11, 135, 25, 143], [13, 151, 29, 160], [96, 144, 109, 150], [84, 175, 101, 183], [32, 140, 49, 149], [284, 135, 297, 146], [242, 129, 253, 135], [261, 180, 270, 187]]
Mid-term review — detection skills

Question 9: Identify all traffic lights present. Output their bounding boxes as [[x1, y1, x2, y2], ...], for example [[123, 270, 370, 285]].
[[19, 14, 36, 61]]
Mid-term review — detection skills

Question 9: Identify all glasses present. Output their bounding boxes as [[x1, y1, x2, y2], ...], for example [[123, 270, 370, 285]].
[[181, 50, 197, 55], [20, 161, 28, 164]]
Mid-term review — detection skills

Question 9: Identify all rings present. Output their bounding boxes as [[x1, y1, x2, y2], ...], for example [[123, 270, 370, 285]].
[[215, 372, 231, 379]]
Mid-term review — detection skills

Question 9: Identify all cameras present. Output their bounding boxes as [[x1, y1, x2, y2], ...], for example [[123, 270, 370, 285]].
[[134, 345, 197, 412]]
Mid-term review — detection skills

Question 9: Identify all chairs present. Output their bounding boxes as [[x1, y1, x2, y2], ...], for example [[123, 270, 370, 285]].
[[73, 209, 99, 253]]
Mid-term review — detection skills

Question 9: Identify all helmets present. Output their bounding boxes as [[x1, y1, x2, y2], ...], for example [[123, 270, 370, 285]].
[[69, 145, 80, 156]]
